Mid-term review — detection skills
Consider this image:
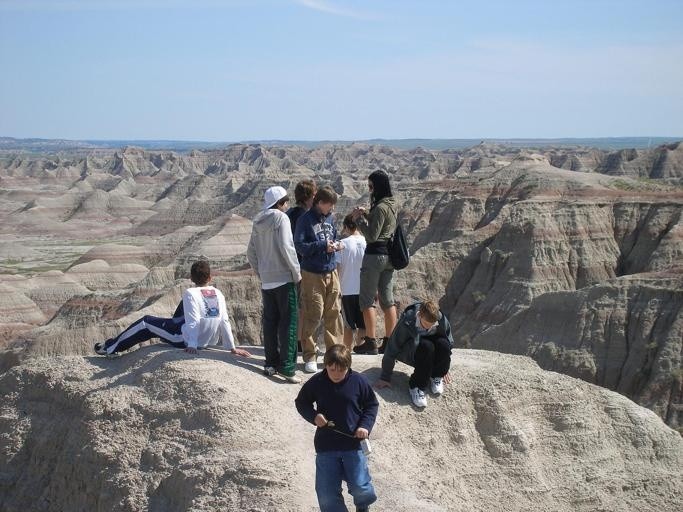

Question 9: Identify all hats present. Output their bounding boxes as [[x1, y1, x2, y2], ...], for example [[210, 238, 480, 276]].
[[264, 186, 287, 210]]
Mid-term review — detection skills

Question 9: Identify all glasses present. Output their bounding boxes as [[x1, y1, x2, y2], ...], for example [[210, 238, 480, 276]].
[[368, 184, 375, 190]]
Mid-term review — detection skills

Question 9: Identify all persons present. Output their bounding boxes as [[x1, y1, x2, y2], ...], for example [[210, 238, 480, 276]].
[[376, 299, 454, 409], [337, 213, 380, 351], [351, 171, 399, 355], [245, 185, 304, 384], [285, 178, 326, 356], [293, 344, 380, 512], [94, 260, 253, 361], [292, 184, 346, 374], [336, 224, 355, 351]]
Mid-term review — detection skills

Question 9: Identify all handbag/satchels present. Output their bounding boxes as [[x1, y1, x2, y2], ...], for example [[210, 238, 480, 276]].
[[386, 224, 409, 270]]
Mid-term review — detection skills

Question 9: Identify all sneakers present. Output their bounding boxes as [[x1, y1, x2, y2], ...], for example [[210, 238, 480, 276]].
[[264, 336, 390, 383], [94, 343, 115, 355], [429, 376, 444, 394], [408, 382, 427, 408]]
